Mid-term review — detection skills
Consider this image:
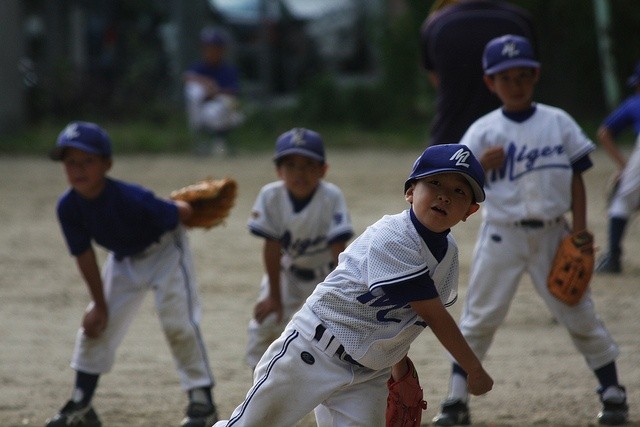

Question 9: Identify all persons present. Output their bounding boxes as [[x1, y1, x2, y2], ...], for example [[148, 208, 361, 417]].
[[419, 1, 531, 139], [435, 33, 628, 424], [180, 25, 243, 137], [47, 122, 217, 426], [591, 73, 640, 270], [243, 128, 353, 372], [210, 144, 493, 426]]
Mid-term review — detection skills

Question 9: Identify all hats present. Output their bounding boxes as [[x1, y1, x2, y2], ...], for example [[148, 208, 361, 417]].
[[272, 128, 324, 161], [405, 144, 485, 202], [482, 35, 539, 76], [51, 122, 111, 161]]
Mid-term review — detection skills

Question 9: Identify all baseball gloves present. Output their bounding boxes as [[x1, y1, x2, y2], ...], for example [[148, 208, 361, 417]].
[[386, 356, 427, 426], [547, 229, 594, 305], [168, 177, 238, 227]]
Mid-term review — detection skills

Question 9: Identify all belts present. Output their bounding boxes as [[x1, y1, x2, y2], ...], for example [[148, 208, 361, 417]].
[[315, 326, 364, 368]]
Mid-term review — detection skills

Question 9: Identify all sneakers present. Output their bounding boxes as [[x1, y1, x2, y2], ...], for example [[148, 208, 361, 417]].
[[433, 399, 470, 425], [46, 401, 101, 426], [597, 387, 628, 425], [180, 401, 218, 427]]
